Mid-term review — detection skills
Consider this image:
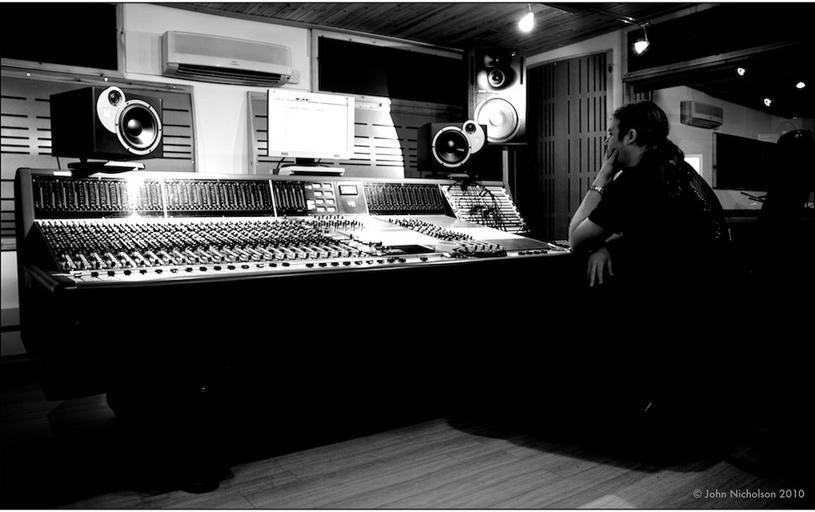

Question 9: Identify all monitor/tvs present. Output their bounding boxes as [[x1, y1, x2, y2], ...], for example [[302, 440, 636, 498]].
[[266, 88, 356, 177]]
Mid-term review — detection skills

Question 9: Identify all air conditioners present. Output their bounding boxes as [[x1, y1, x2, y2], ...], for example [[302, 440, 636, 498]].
[[679, 100, 723, 128], [159, 31, 302, 87]]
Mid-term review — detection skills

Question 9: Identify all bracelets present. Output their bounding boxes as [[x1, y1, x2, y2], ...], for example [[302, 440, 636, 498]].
[[588, 182, 605, 198]]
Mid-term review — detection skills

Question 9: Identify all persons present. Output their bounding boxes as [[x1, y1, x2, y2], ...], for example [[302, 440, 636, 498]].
[[568, 102, 730, 318]]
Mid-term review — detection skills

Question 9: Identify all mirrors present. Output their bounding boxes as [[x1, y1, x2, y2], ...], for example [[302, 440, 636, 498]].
[[622, 39, 814, 212]]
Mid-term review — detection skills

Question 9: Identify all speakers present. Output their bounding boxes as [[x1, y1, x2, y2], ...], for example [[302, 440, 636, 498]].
[[416, 122, 487, 172], [49, 85, 164, 162], [466, 47, 527, 144]]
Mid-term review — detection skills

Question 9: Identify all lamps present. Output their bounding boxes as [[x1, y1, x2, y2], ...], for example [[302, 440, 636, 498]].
[[517, 4, 537, 38], [635, 24, 651, 55]]
